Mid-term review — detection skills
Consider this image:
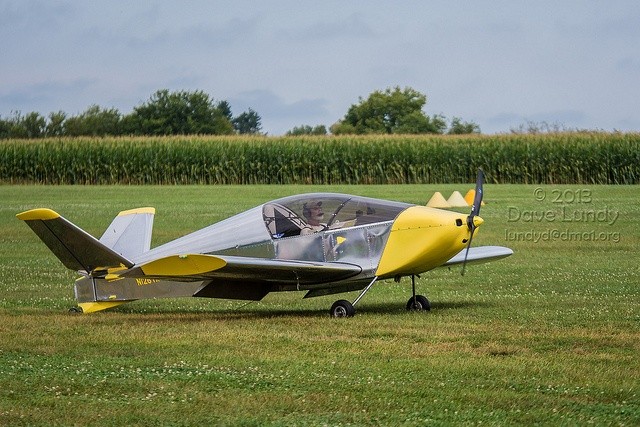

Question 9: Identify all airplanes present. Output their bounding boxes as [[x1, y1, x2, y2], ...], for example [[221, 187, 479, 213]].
[[15, 169, 514, 319]]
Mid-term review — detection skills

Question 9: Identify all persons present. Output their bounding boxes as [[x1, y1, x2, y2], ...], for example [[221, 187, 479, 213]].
[[300, 201, 329, 235]]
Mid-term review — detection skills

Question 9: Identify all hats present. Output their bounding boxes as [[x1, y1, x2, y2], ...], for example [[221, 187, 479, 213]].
[[305, 201, 322, 207]]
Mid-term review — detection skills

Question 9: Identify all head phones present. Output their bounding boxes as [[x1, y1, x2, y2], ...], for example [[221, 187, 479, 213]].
[[302, 203, 310, 218]]
